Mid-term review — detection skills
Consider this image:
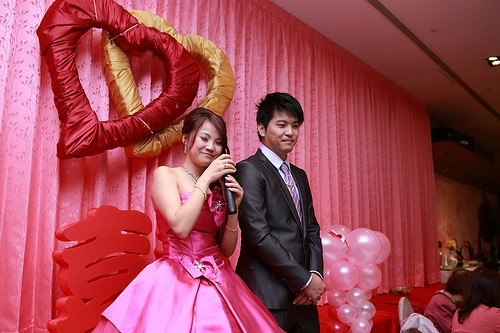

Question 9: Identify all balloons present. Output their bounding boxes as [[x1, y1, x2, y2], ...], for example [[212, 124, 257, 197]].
[[318, 223, 391, 333]]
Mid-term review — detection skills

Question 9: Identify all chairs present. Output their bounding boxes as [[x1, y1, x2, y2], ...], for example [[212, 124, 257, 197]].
[[400, 313, 440, 333], [398, 297, 414, 328]]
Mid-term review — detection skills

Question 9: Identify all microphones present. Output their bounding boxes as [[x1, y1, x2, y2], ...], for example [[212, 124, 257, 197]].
[[222, 174, 237, 214]]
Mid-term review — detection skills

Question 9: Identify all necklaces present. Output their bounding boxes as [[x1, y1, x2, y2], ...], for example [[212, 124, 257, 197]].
[[182, 165, 197, 184]]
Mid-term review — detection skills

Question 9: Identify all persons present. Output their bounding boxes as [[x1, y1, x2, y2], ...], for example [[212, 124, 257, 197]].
[[424, 266, 500, 333], [232, 92, 327, 333], [88, 108, 287, 333]]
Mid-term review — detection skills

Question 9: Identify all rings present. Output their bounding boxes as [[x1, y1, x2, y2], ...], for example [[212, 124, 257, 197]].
[[224, 164, 227, 168]]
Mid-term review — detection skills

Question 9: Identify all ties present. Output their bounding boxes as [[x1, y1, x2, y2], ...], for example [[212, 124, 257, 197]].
[[279, 162, 302, 224]]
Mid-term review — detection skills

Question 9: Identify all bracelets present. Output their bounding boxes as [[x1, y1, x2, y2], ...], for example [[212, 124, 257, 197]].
[[225, 223, 239, 232], [194, 185, 208, 200]]
